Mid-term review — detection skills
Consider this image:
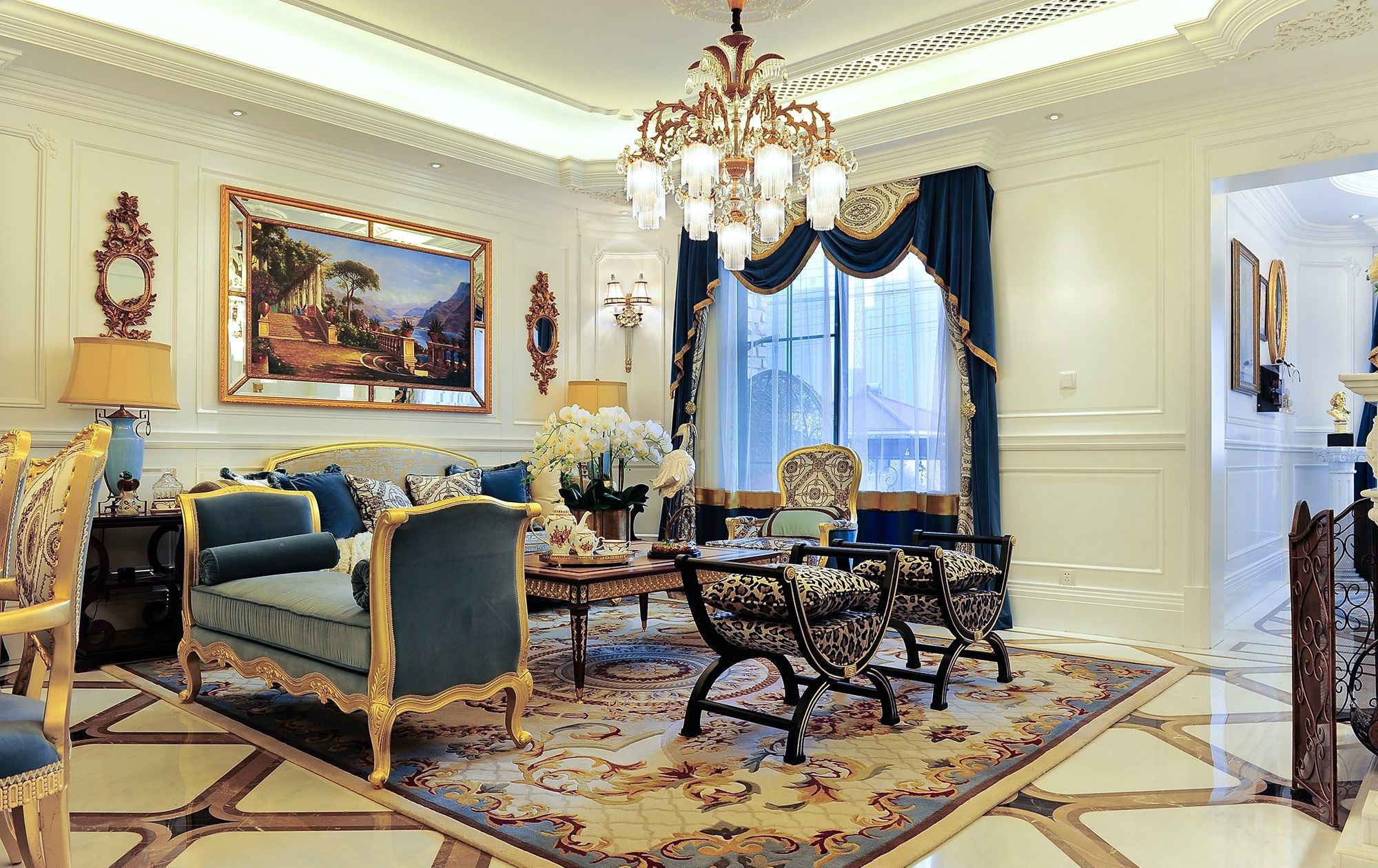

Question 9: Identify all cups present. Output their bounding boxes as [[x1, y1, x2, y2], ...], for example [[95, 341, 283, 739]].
[[601, 539, 631, 553]]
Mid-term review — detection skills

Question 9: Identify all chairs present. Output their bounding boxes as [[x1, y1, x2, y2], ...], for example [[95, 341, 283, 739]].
[[0, 424, 113, 868], [0, 429, 34, 611], [705, 444, 863, 574]]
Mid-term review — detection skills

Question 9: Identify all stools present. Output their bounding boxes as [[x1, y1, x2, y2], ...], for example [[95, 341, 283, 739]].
[[676, 542, 905, 767], [833, 527, 1016, 712]]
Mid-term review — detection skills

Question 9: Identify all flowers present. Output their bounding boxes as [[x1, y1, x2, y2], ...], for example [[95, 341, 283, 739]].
[[522, 407, 675, 513]]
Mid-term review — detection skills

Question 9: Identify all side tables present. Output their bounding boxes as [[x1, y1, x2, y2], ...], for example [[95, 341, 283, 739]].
[[72, 511, 187, 674]]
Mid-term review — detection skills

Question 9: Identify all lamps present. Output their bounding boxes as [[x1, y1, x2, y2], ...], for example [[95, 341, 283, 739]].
[[603, 271, 652, 374], [565, 378, 632, 481], [57, 335, 181, 516], [615, 0, 858, 274]]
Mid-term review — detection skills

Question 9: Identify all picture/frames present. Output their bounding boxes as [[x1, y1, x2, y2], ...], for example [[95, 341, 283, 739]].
[[216, 182, 493, 416], [1258, 274, 1269, 341], [1230, 238, 1263, 397], [1266, 258, 1288, 363]]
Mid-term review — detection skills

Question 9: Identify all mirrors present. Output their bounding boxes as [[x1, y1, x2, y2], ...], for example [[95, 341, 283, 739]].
[[524, 271, 560, 395], [93, 190, 159, 342]]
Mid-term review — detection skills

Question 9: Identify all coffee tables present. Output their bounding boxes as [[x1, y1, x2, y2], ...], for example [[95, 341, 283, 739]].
[[524, 538, 782, 700]]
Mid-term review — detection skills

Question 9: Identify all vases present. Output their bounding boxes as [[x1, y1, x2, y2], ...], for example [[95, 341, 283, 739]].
[[570, 507, 631, 543]]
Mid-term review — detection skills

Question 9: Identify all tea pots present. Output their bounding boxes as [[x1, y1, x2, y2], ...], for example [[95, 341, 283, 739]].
[[529, 498, 606, 557]]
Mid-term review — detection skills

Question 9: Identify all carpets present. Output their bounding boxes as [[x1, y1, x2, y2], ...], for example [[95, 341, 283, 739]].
[[96, 593, 1194, 868]]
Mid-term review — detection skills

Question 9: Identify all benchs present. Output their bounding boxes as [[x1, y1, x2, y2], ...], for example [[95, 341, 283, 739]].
[[174, 484, 542, 788]]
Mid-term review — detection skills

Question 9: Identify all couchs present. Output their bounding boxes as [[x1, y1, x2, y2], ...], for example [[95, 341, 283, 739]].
[[187, 438, 551, 551]]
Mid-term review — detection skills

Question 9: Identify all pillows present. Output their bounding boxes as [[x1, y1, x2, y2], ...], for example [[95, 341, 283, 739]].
[[270, 464, 367, 539], [446, 460, 531, 504], [219, 467, 288, 489], [756, 506, 850, 540], [700, 563, 880, 621], [529, 456, 572, 502], [849, 545, 1000, 593], [198, 531, 340, 586], [350, 559, 371, 612], [344, 473, 413, 532], [404, 468, 483, 507]]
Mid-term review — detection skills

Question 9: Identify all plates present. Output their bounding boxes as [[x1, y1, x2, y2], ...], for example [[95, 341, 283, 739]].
[[595, 550, 630, 555]]
